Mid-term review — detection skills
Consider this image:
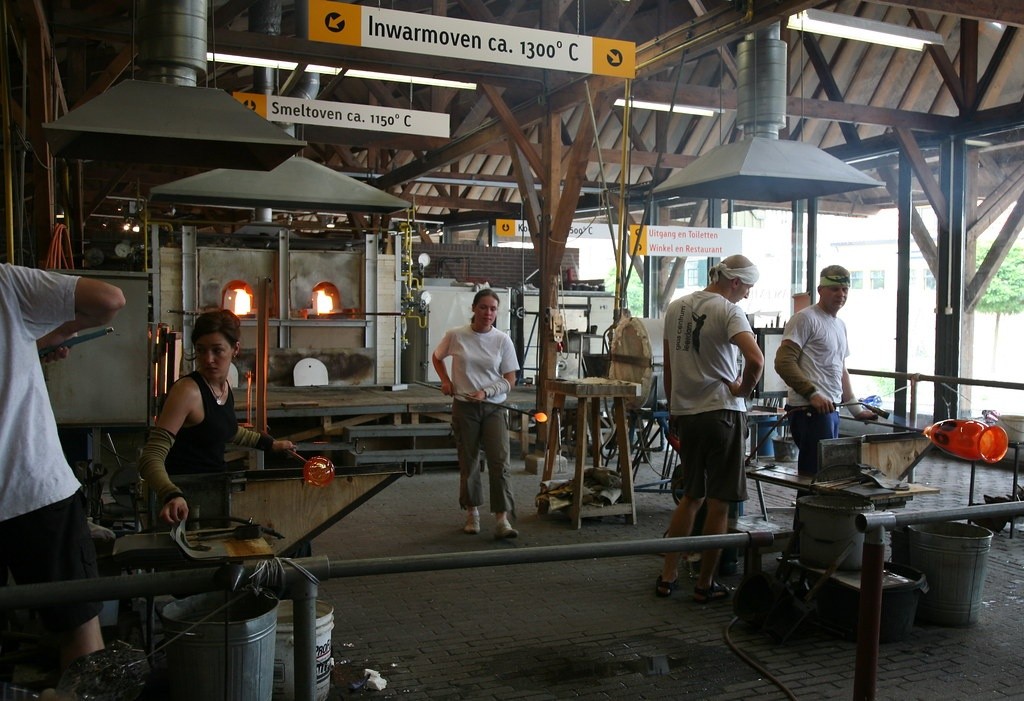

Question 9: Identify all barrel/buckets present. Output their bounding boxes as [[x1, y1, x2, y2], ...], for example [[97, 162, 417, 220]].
[[813, 561, 927, 645], [772, 435, 799, 462], [154, 590, 280, 701], [272, 599, 335, 701], [903, 521, 994, 629], [998, 416, 1024, 444], [794, 495, 875, 571]]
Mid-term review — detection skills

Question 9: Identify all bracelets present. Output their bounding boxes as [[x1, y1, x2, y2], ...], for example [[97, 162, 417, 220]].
[[844, 396, 864, 417]]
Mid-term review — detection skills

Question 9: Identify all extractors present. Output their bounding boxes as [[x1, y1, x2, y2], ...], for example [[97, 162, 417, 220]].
[[649, 1, 887, 204], [38, 0, 308, 172], [146, 1, 414, 215]]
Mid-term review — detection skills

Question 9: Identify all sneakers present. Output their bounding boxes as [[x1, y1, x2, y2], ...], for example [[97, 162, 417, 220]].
[[494, 523, 519, 538], [464, 513, 480, 533]]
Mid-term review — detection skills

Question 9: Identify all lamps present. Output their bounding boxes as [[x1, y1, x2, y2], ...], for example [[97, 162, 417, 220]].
[[787, 9, 945, 52], [615, 99, 726, 116]]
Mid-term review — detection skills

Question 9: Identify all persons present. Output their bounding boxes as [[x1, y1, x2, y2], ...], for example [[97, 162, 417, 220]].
[[656, 254, 764, 603], [774, 266, 878, 532], [432, 289, 520, 539], [137, 310, 298, 532], [0, 262, 126, 662]]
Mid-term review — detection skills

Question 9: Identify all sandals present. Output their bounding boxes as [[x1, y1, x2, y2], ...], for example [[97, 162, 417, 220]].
[[693, 580, 731, 603], [656, 575, 679, 598]]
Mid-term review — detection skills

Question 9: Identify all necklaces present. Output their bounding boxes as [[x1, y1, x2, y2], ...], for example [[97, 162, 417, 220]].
[[206, 378, 225, 405]]
